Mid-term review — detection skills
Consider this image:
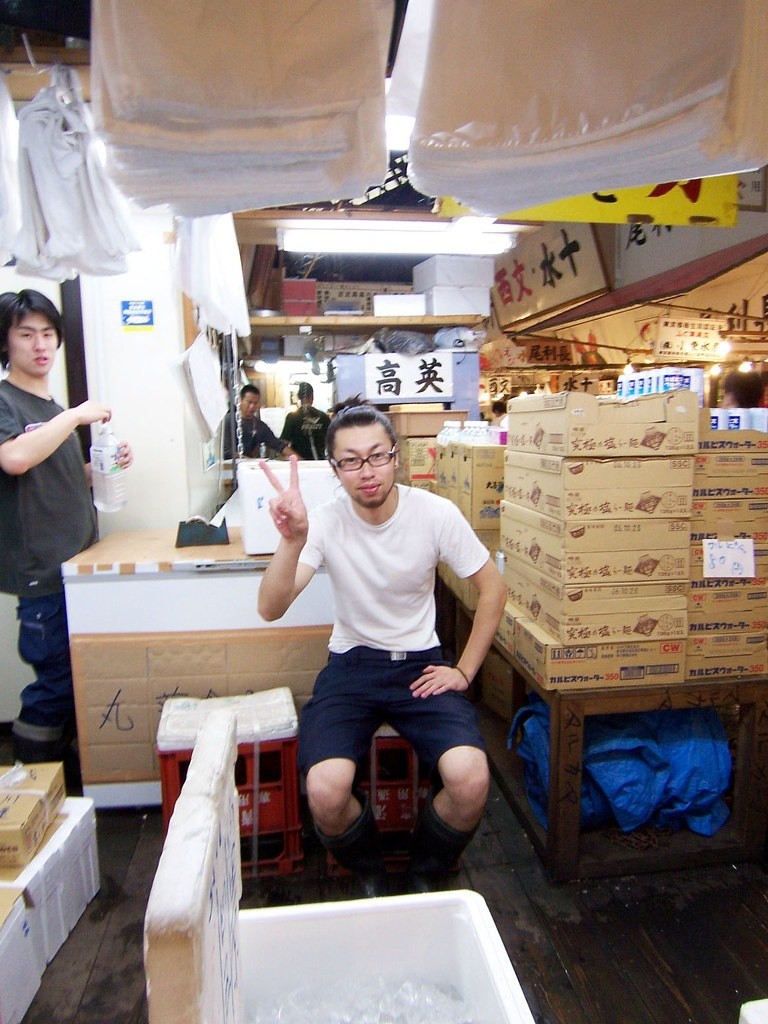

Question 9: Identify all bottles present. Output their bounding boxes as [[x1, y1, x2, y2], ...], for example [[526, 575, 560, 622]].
[[519, 380, 551, 397], [90, 421, 127, 511], [435, 420, 508, 445], [259, 442, 266, 459]]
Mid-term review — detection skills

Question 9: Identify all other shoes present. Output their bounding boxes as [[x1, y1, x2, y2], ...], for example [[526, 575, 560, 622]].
[[12, 741, 83, 796]]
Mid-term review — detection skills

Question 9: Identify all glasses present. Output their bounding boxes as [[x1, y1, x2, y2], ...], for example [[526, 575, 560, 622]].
[[330, 442, 398, 471]]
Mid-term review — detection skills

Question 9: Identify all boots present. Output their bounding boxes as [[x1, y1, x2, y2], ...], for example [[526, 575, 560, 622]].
[[315, 789, 481, 898]]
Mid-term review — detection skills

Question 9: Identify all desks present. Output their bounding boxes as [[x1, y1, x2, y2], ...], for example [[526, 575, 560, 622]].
[[447, 590, 767, 887], [64, 524, 349, 806]]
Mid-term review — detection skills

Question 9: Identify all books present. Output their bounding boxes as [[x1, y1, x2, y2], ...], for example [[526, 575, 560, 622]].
[[176, 332, 229, 444]]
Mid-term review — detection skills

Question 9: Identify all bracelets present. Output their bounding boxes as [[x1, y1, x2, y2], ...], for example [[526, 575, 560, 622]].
[[452, 666, 470, 687]]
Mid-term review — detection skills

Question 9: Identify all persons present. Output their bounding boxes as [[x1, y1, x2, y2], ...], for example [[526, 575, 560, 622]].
[[0, 289, 133, 797], [223, 385, 303, 460], [279, 382, 331, 460], [257, 405, 508, 897], [723, 371, 762, 408]]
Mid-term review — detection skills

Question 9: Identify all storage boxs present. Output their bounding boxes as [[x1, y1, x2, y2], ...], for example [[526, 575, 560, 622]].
[[0, 254, 768, 1023]]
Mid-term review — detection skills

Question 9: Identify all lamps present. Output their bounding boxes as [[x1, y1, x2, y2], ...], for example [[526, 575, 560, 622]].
[[274, 225, 518, 256]]
